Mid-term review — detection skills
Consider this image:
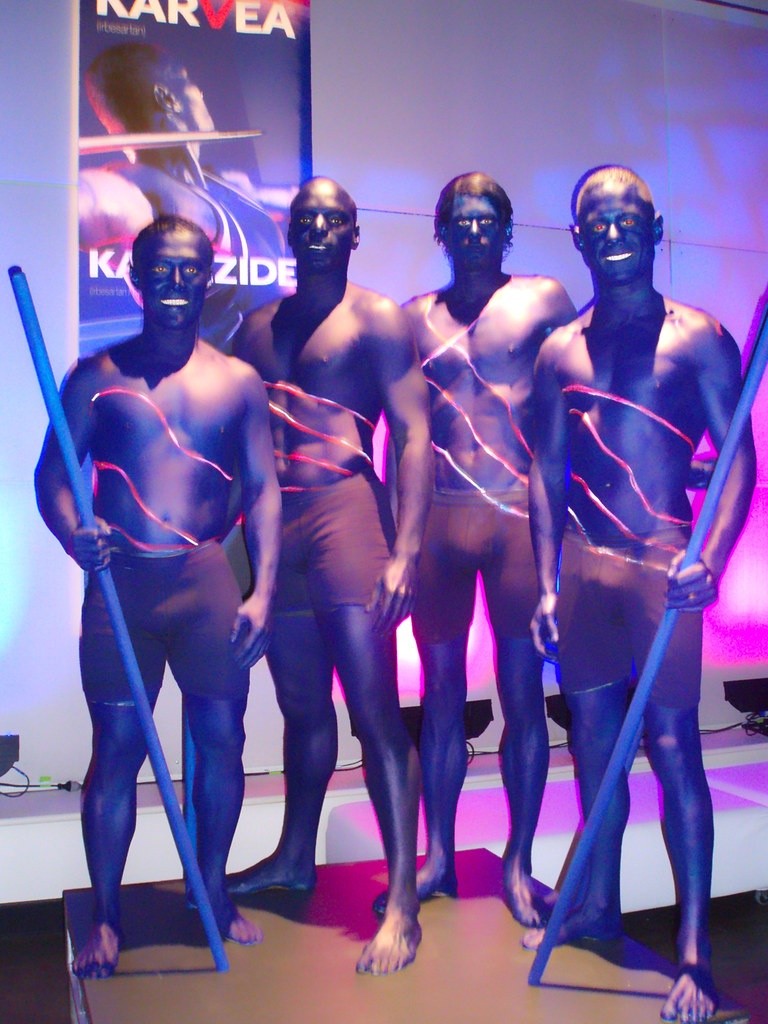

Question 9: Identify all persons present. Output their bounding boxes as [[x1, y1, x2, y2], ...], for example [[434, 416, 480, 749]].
[[34, 215, 283, 978], [373, 172, 577, 929], [520, 168, 757, 1024], [76, 43, 287, 356], [232, 176, 434, 976]]
